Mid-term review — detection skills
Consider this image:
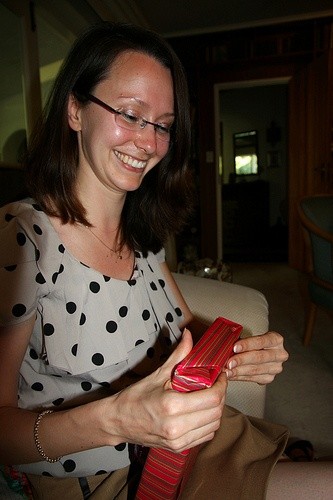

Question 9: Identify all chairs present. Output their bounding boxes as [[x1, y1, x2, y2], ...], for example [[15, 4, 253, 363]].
[[293, 188, 333, 347]]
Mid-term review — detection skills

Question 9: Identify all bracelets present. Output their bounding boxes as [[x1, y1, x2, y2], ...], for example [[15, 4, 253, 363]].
[[34, 411, 64, 463]]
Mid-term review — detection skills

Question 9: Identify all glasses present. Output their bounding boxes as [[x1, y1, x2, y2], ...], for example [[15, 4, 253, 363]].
[[88, 94, 180, 143]]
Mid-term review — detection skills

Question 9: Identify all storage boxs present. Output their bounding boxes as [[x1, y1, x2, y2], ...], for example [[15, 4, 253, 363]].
[[135, 316, 243, 500]]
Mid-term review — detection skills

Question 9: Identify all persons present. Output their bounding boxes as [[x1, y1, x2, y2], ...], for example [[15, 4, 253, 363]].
[[0, 25, 333, 500]]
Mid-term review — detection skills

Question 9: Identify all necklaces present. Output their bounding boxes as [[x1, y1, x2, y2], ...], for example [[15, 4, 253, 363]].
[[86, 226, 125, 260]]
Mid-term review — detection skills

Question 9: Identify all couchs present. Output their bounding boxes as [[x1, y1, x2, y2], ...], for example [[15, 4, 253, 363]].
[[171, 273, 269, 420]]
